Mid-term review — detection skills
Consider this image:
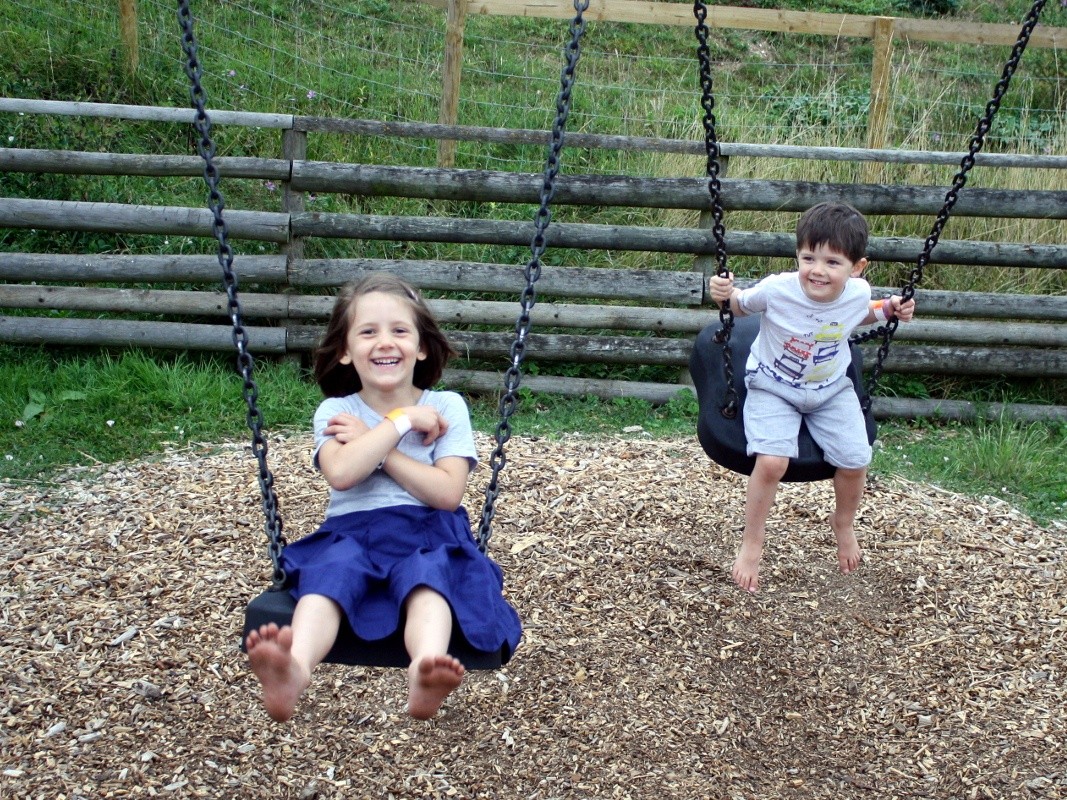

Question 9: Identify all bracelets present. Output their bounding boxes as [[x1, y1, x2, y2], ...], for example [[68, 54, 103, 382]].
[[874, 298, 891, 321], [384, 407, 413, 439]]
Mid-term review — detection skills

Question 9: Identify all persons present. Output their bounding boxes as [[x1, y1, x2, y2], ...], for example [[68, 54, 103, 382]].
[[242, 273, 521, 722], [709, 201, 915, 593]]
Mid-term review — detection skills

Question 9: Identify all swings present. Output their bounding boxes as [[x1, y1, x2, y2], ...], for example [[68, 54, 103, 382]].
[[174, 2, 586, 673], [692, 2, 1046, 483]]
